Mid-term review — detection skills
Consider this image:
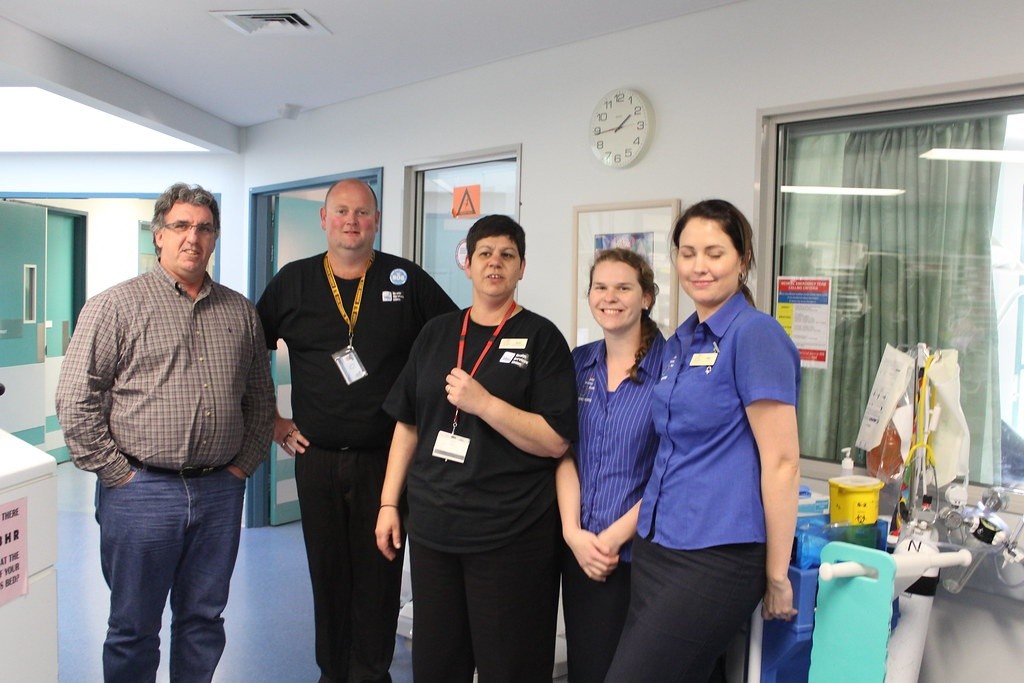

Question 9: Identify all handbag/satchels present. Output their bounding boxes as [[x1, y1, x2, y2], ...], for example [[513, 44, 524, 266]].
[[927, 349, 970, 488]]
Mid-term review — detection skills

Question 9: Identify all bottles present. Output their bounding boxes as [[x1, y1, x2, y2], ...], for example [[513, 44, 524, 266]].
[[836, 448, 855, 476]]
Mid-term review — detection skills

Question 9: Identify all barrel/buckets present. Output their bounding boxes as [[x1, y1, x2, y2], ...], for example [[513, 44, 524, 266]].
[[829, 475, 885, 525]]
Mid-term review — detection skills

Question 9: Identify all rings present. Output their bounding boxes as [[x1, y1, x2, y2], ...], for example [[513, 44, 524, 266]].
[[281, 442, 286, 447], [283, 435, 288, 442], [289, 430, 295, 436]]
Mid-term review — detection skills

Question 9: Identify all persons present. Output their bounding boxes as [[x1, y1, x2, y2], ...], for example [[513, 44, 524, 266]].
[[781, 242, 942, 468], [374, 214, 577, 683], [607, 200, 800, 683], [555, 248, 666, 683], [56, 183, 275, 683], [256, 180, 460, 683]]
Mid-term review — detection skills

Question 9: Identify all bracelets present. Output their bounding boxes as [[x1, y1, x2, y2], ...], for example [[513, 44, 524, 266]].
[[379, 504, 399, 509]]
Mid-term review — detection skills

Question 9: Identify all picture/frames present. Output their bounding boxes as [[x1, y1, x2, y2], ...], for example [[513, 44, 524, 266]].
[[571, 198, 681, 354]]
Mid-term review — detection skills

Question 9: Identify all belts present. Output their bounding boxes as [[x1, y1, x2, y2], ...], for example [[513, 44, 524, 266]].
[[120, 451, 235, 478]]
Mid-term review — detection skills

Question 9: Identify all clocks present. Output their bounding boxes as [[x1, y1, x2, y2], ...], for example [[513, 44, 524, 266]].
[[589, 88, 657, 170]]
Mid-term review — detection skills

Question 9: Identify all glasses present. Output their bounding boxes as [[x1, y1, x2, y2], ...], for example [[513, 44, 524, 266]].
[[165, 221, 218, 233]]
